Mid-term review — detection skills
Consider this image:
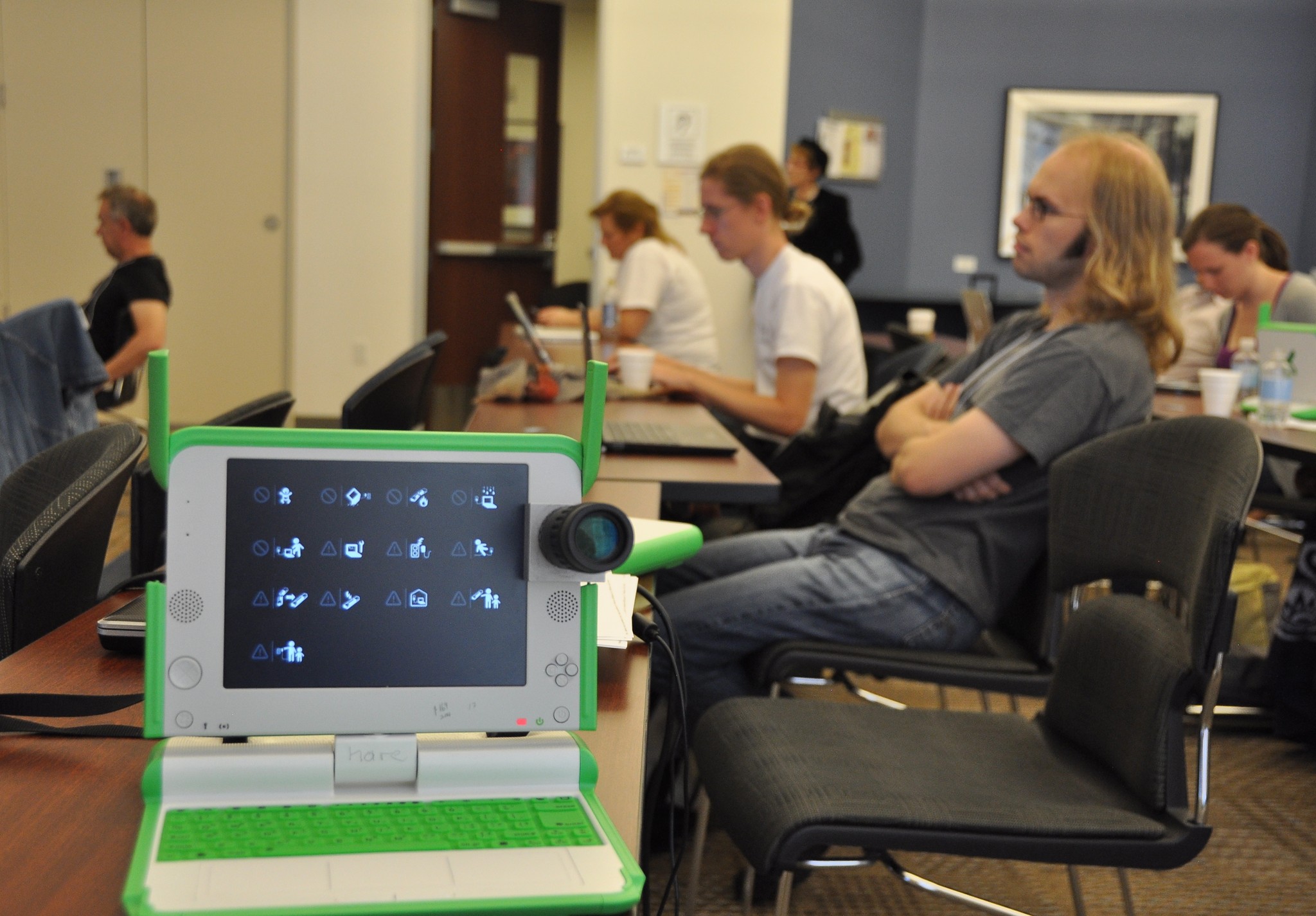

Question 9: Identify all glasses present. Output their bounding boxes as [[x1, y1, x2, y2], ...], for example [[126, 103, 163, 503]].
[[1019, 190, 1091, 224]]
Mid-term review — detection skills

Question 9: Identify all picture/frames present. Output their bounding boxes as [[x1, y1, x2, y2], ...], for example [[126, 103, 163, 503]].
[[995, 87, 1221, 267]]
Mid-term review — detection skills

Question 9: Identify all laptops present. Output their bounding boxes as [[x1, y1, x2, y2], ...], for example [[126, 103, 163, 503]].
[[963, 292, 993, 338], [96, 593, 145, 652], [122, 346, 645, 916], [1241, 302, 1316, 421], [612, 516, 704, 578], [507, 290, 737, 459]]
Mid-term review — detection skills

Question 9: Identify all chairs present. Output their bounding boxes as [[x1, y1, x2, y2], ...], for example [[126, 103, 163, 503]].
[[341, 348, 436, 431], [0, 423, 148, 658], [392, 331, 448, 431], [684, 329, 1265, 916], [97, 390, 295, 601]]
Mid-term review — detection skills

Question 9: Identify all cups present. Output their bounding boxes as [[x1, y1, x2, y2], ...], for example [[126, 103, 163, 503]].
[[1197, 367, 1242, 418], [907, 308, 936, 337], [616, 349, 655, 392]]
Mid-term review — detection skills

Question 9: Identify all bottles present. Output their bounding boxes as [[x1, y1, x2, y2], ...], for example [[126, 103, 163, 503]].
[[1257, 348, 1296, 430], [1230, 335, 1263, 416]]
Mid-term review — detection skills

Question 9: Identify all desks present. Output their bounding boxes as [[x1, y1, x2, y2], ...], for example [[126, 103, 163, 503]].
[[863, 332, 1316, 458], [0, 321, 781, 916]]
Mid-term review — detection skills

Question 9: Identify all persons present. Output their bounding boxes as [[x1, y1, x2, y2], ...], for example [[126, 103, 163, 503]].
[[782, 134, 863, 283], [536, 189, 718, 374], [76, 186, 171, 407], [599, 140, 867, 541], [1183, 204, 1316, 498], [637, 125, 1185, 856]]
